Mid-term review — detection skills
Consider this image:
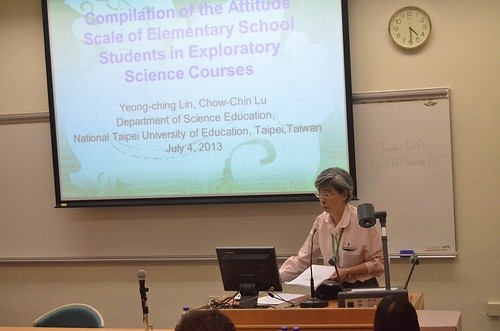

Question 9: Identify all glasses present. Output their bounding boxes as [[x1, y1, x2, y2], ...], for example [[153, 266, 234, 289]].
[[314, 191, 343, 200]]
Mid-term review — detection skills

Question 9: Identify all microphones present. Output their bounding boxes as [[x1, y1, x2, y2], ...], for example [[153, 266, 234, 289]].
[[138, 270, 146, 308], [300, 228, 329, 308]]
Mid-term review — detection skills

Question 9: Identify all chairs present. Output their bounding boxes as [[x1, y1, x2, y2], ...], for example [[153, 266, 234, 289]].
[[32, 301, 106, 328]]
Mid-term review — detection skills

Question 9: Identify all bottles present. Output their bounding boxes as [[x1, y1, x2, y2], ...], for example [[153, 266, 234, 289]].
[[182, 306, 190, 317]]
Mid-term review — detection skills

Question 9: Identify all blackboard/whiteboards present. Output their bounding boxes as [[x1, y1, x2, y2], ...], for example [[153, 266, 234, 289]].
[[0, 87, 457, 260]]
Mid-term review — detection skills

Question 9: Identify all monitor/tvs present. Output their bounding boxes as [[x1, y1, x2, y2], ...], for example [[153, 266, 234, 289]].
[[215, 246, 283, 308]]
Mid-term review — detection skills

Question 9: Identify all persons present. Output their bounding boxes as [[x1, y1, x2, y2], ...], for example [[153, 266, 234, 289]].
[[277, 167, 390, 288], [373, 295, 420, 331], [175, 309, 236, 331]]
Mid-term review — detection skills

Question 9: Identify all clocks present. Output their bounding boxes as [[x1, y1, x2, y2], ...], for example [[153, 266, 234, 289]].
[[388, 6, 432, 51]]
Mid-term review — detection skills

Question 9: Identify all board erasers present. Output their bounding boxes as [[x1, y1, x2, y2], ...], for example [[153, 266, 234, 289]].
[[399, 250, 415, 256]]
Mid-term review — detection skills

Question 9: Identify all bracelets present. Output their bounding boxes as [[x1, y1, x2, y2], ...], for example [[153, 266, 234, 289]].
[[347, 268, 350, 278]]
